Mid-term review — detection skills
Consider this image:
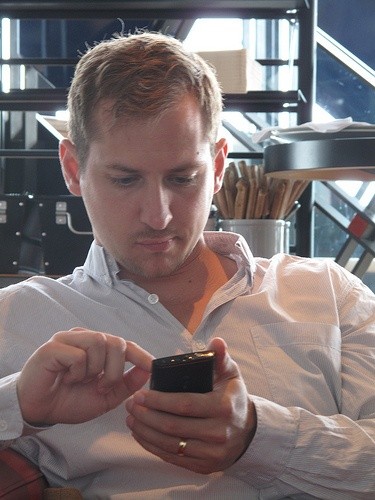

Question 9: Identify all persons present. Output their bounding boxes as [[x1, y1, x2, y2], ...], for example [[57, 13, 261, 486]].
[[0, 32, 375, 500]]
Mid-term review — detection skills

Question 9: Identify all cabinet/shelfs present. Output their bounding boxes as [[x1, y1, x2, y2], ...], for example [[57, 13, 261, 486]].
[[0, 0, 318, 288]]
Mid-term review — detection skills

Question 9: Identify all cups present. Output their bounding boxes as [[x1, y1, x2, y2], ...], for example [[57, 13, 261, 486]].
[[217, 217, 291, 259]]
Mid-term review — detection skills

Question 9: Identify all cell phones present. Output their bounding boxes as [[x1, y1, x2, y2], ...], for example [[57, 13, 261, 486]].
[[150, 350, 217, 392]]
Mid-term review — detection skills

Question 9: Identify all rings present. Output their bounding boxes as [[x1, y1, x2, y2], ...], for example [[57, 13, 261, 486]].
[[177, 438, 188, 457]]
